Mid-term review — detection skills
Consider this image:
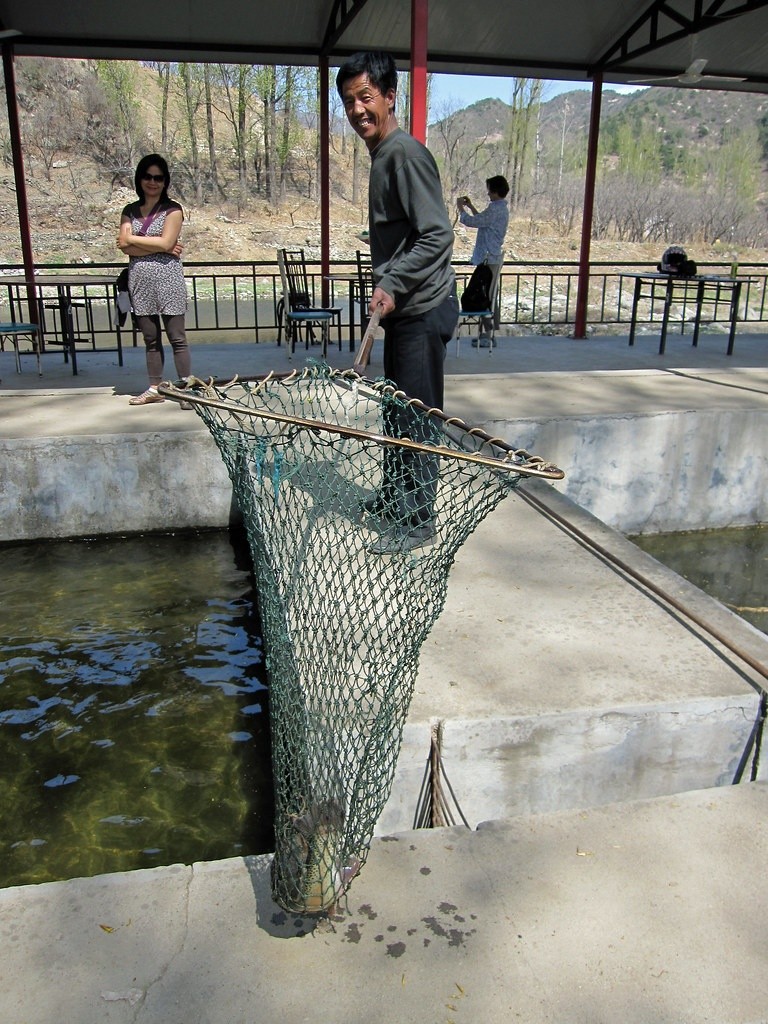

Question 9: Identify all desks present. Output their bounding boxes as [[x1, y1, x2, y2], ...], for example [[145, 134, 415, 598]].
[[0, 275, 137, 376], [324, 271, 469, 364], [619, 272, 759, 356]]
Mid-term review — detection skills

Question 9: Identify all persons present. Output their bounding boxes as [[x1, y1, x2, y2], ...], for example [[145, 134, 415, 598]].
[[116, 155, 192, 412], [337, 49, 460, 555], [457, 176, 510, 347]]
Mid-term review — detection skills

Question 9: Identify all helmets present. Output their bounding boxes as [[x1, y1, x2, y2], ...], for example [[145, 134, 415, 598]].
[[661, 247, 687, 275]]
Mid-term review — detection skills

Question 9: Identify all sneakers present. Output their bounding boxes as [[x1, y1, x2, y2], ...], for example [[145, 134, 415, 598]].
[[180, 387, 194, 410], [129, 390, 165, 404]]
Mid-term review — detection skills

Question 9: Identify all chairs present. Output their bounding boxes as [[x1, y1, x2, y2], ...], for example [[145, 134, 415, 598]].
[[276, 250, 331, 357], [454, 250, 508, 352], [0, 323, 44, 378], [355, 250, 383, 366], [285, 249, 343, 352]]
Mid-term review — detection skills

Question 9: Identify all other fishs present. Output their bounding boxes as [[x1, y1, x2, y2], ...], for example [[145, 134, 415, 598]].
[[271, 799, 360, 912]]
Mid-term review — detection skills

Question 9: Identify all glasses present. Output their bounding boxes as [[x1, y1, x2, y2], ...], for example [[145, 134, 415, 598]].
[[143, 173, 164, 182]]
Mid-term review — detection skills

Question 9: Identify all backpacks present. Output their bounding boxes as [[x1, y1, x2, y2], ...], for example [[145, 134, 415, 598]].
[[462, 259, 492, 312]]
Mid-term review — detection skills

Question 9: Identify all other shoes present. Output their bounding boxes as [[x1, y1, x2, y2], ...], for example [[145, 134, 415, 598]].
[[472, 334, 496, 347], [359, 500, 436, 527], [371, 524, 437, 555]]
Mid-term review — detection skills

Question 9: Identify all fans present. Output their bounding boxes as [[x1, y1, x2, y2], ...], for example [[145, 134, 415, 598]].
[[624, 31, 747, 86]]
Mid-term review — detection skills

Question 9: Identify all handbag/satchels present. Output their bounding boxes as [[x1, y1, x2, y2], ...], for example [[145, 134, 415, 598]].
[[678, 261, 698, 277], [277, 294, 309, 326], [117, 269, 129, 291]]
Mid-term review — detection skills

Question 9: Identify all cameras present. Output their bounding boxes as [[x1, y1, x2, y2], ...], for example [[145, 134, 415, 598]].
[[463, 197, 467, 204]]
